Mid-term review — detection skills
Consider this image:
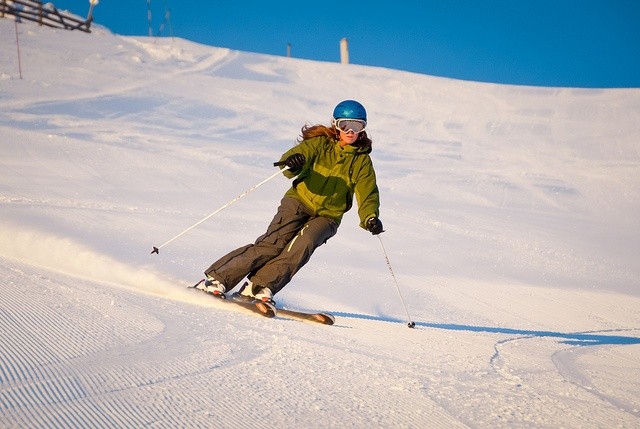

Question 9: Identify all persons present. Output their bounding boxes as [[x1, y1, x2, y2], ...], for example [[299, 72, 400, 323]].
[[194, 100, 383, 315]]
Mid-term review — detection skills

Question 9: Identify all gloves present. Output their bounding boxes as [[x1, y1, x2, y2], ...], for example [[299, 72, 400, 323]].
[[286, 153, 305, 169], [366, 217, 384, 235]]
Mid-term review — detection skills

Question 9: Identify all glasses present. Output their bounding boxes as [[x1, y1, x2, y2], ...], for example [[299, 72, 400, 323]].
[[332, 119, 366, 133]]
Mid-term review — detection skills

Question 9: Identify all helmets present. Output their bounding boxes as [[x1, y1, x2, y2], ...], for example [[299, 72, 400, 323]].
[[334, 101, 366, 118]]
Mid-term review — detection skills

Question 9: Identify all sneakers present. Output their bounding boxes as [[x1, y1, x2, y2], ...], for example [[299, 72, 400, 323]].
[[202, 277, 226, 296], [239, 282, 274, 306]]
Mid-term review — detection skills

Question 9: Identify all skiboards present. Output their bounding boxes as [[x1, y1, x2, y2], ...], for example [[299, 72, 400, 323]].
[[188, 286, 335, 326]]
